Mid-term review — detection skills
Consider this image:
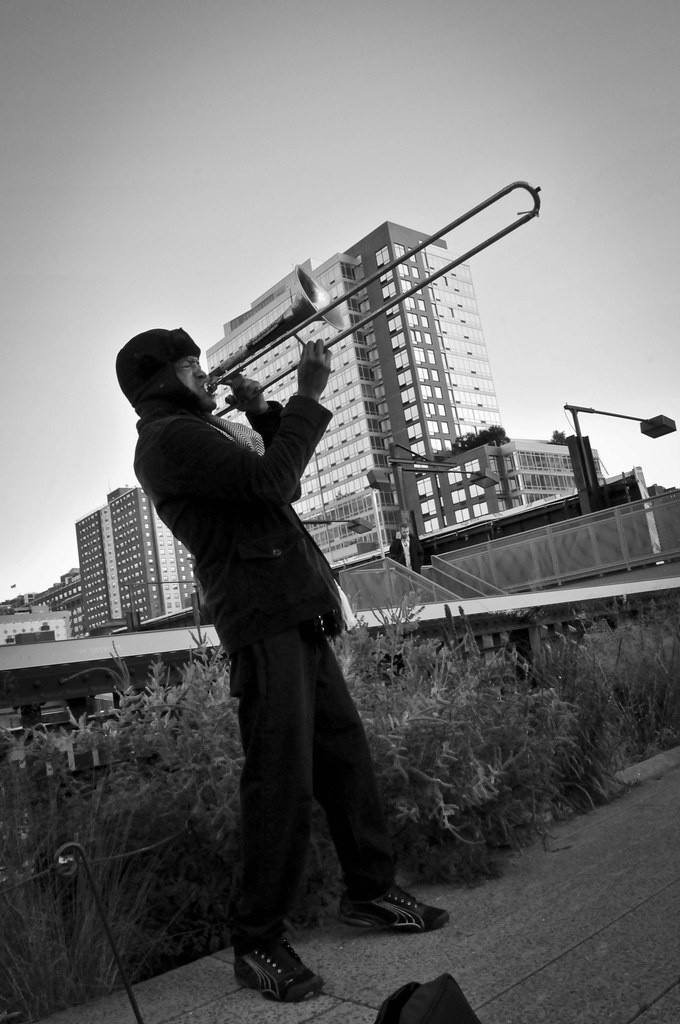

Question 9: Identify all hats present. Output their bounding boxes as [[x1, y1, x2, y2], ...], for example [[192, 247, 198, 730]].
[[116, 330, 200, 419]]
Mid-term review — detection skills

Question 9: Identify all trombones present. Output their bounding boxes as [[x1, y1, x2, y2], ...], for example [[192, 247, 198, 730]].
[[204, 178, 543, 417]]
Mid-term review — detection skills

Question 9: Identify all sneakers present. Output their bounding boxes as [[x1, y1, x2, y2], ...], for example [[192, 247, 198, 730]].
[[338, 882, 448, 933], [234, 935, 323, 1002]]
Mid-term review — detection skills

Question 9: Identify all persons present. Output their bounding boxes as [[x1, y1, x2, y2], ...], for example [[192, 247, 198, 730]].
[[114, 325, 447, 1003], [390, 523, 426, 602]]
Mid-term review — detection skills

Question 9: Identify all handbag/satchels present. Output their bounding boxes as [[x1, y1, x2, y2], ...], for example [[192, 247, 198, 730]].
[[374, 974, 480, 1024]]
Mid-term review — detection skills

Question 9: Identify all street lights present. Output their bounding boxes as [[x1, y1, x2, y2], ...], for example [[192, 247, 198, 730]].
[[563, 402, 676, 513], [365, 442, 500, 524]]
